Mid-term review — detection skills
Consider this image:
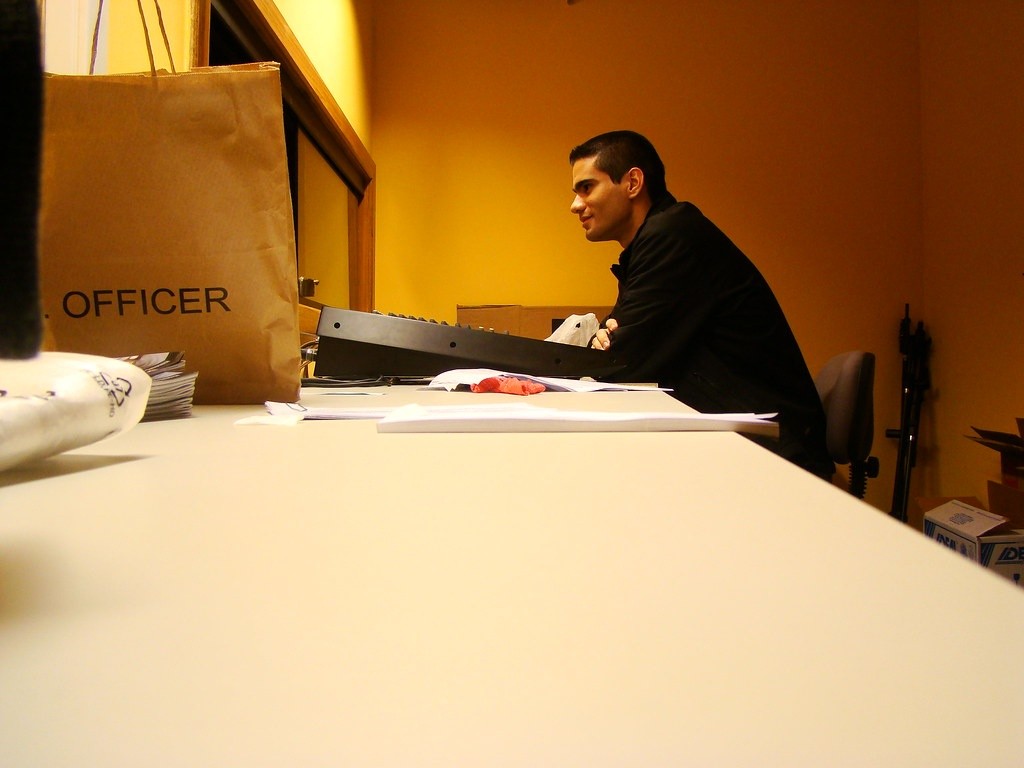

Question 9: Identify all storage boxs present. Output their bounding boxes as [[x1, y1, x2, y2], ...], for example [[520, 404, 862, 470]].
[[457, 304, 614, 340], [914, 416, 1024, 588]]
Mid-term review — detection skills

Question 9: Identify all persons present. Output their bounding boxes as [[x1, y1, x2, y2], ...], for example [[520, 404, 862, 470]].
[[569, 130, 836, 484]]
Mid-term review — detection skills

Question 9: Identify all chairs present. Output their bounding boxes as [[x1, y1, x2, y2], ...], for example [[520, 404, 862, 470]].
[[812, 348, 880, 498]]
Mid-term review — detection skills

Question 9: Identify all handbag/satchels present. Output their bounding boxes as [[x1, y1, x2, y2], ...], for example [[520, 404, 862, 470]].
[[39, 0, 304, 405]]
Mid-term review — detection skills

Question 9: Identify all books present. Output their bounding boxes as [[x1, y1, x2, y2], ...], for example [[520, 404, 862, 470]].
[[112, 350, 200, 423]]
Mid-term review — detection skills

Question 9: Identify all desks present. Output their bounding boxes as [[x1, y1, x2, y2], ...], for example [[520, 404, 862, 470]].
[[0, 383, 1024, 768]]
